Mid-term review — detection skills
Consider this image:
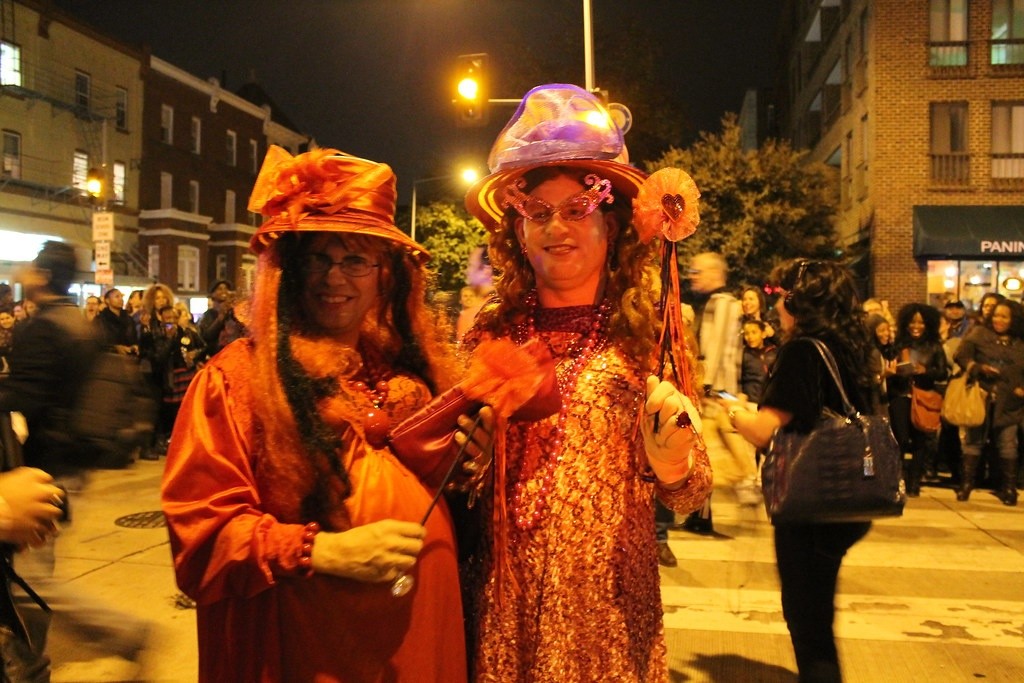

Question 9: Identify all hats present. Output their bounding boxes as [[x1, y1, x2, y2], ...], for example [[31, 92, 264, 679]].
[[463, 81, 702, 245], [246, 145, 431, 266]]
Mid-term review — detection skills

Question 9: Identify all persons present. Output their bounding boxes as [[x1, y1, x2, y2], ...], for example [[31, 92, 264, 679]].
[[0, 467, 66, 622], [91, 280, 246, 463], [457, 286, 484, 343], [713, 257, 886, 683], [160, 144, 496, 683], [862, 292, 1024, 505], [0, 239, 107, 476], [457, 82, 712, 683], [656, 252, 783, 567]]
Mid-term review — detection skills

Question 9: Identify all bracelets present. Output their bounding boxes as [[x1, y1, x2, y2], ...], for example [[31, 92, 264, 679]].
[[296, 521, 321, 579], [728, 406, 747, 429]]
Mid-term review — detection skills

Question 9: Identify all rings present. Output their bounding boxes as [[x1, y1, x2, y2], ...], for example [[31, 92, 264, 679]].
[[390, 571, 414, 597], [674, 412, 692, 428], [472, 451, 484, 459]]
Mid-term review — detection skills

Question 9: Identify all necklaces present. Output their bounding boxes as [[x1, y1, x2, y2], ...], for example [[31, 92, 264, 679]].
[[515, 289, 612, 530], [347, 335, 391, 437]]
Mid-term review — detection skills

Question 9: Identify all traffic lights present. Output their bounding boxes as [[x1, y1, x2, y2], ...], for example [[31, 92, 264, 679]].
[[86, 166, 104, 204], [452, 53, 489, 128]]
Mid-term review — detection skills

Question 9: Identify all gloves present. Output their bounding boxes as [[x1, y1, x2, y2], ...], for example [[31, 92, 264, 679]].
[[640, 374, 702, 481]]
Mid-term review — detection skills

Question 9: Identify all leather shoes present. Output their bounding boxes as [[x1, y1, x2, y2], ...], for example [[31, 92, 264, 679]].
[[655, 541, 678, 567]]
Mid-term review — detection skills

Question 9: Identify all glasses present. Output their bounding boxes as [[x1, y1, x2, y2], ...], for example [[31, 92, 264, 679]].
[[296, 249, 383, 277], [500, 173, 615, 223]]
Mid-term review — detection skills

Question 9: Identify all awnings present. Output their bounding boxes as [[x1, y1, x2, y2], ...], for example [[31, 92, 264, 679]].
[[912, 205, 1024, 261]]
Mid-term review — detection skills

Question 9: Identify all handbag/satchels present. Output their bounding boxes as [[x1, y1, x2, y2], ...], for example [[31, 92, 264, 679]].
[[760, 337, 906, 525], [386, 336, 561, 492], [910, 384, 942, 433], [942, 358, 988, 429]]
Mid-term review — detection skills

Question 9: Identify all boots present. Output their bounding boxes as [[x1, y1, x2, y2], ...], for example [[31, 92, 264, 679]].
[[998, 456, 1019, 505], [139, 435, 159, 460], [154, 434, 169, 455], [957, 454, 978, 500], [908, 440, 927, 497]]
